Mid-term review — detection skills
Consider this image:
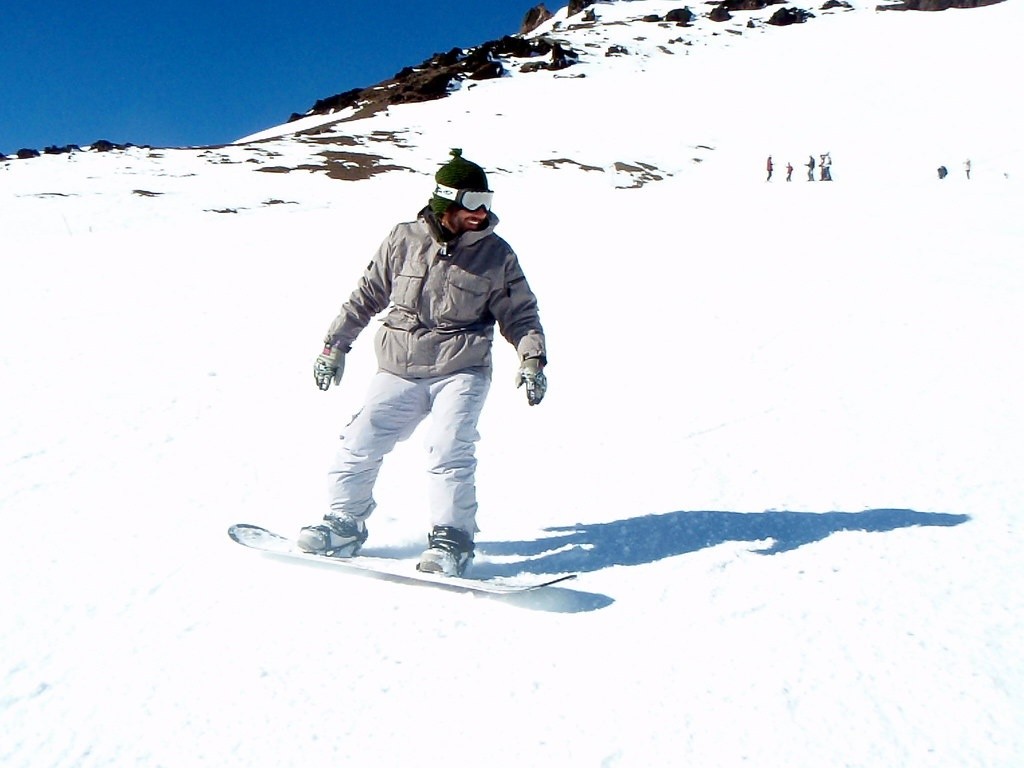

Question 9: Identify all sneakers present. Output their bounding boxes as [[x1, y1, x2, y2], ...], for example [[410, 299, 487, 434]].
[[297, 511, 368, 557], [416, 525, 475, 578]]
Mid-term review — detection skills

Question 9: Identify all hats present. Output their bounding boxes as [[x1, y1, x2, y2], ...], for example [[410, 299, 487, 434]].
[[429, 149, 488, 224]]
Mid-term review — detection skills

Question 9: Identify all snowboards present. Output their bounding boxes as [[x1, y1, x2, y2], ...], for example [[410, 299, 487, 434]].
[[227, 521, 578, 597]]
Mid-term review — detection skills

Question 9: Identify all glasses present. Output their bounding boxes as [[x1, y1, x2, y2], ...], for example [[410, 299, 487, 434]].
[[459, 189, 494, 213]]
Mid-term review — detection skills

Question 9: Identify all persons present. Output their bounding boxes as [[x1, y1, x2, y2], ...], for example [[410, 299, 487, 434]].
[[299, 148, 546, 577], [786, 162, 793, 182], [767, 157, 773, 180], [804, 155, 815, 181], [964, 158, 970, 179], [938, 166, 947, 178], [819, 153, 832, 181]]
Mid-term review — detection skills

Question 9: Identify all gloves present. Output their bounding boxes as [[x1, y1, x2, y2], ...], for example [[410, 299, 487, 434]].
[[314, 343, 345, 391], [515, 357, 547, 407]]
